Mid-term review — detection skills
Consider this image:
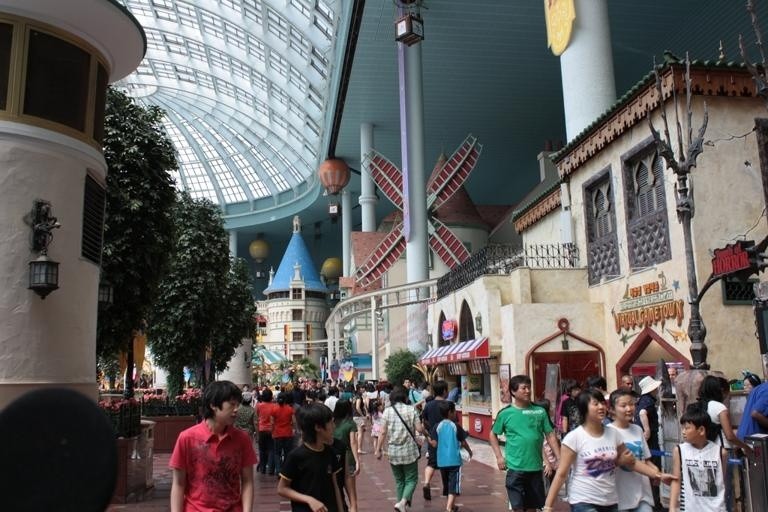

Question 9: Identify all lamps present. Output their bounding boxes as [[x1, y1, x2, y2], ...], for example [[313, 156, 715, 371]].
[[24, 196, 61, 300]]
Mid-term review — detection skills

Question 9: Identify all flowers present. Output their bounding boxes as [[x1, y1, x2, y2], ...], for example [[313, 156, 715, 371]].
[[133, 386, 169, 417], [176, 386, 205, 417], [98, 398, 142, 439]]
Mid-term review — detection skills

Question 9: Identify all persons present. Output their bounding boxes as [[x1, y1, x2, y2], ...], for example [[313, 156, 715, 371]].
[[489, 368, 768, 512], [169, 379, 473, 512]]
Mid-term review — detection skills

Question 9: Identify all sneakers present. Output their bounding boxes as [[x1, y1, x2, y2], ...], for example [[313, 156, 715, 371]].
[[452, 505, 464, 512], [260, 466, 265, 475], [423, 483, 432, 501], [267, 467, 273, 475], [393, 502, 408, 512]]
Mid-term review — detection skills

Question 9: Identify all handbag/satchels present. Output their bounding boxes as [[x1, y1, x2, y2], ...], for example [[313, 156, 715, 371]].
[[417, 444, 422, 460]]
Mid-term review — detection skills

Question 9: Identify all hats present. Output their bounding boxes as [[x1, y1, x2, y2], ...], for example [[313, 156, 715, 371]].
[[638, 374, 664, 397]]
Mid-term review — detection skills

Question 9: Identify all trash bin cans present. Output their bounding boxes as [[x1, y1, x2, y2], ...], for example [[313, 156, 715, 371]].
[[140, 419, 156, 489]]
